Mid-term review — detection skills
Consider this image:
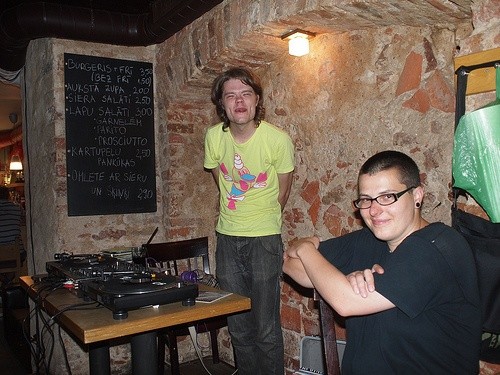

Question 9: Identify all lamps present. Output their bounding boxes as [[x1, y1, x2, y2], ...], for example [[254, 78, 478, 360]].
[[281, 29, 316, 56], [8, 114, 23, 170]]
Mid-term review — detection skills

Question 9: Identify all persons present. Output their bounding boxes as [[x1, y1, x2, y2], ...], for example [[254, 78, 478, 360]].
[[204, 67, 295, 375], [0, 184, 27, 288], [281, 150, 482, 375]]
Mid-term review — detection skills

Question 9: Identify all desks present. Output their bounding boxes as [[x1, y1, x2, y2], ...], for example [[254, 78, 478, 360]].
[[18, 274, 251, 375]]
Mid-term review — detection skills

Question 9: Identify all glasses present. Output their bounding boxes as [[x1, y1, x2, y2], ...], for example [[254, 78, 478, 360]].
[[353, 185, 416, 209]]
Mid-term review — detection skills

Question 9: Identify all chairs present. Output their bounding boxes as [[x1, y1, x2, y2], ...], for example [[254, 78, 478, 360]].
[[0, 238, 21, 289], [142, 236, 228, 375]]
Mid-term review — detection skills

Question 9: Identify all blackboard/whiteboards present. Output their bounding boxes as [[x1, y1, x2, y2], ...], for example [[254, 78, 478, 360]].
[[62, 51, 158, 217]]
